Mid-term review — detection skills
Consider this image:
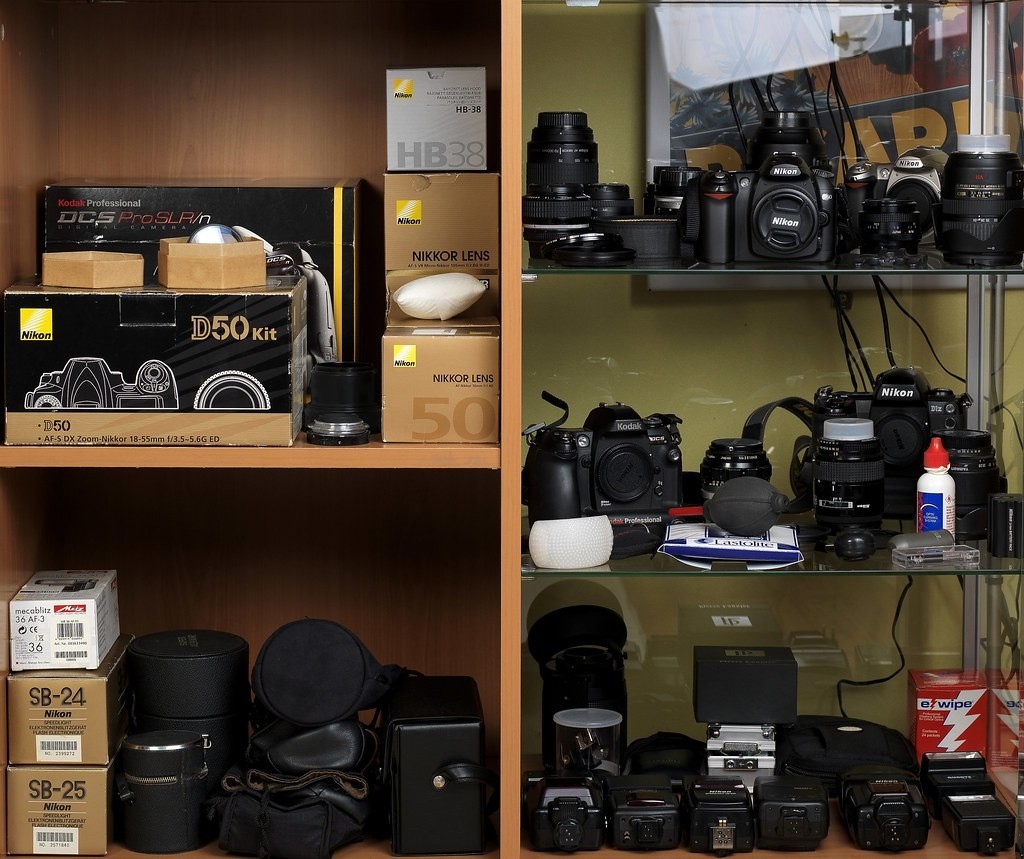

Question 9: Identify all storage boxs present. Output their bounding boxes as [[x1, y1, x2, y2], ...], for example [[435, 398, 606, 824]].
[[7, 736, 127, 855], [9, 569, 120, 670], [386, 68, 488, 172], [7, 636, 135, 765], [1, 276, 308, 449], [385, 172, 501, 271], [42, 178, 371, 363], [907, 670, 989, 764], [381, 329, 500, 444], [386, 273, 500, 328]]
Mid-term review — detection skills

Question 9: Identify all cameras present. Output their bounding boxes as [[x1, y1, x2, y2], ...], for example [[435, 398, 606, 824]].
[[530, 109, 1024, 547], [522, 603, 1017, 856]]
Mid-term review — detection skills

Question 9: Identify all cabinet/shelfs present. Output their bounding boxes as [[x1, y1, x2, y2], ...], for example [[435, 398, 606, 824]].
[[0, 0, 1024, 859]]
